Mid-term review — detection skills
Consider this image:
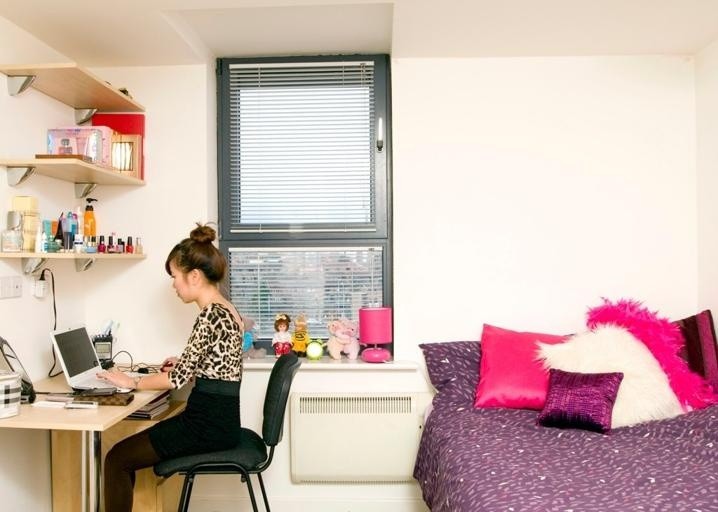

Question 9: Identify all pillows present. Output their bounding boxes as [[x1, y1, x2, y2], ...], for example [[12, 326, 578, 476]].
[[418, 298, 717, 433]]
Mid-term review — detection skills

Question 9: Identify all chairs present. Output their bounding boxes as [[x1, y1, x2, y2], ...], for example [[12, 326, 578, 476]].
[[151, 347, 300, 512]]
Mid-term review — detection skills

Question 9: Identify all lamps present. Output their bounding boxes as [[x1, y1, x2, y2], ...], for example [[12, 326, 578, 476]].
[[356, 304, 394, 363]]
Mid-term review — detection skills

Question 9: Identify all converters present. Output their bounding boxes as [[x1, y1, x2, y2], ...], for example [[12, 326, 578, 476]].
[[35, 280, 49, 297]]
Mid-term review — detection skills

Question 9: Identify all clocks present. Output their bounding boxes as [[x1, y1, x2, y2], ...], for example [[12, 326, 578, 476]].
[[303, 337, 326, 362]]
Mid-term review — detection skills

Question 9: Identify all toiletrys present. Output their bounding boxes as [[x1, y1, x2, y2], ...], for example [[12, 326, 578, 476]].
[[1, 195, 143, 254]]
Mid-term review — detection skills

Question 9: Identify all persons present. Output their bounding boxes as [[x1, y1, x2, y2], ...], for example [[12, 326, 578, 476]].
[[270, 313, 293, 358], [97, 222, 245, 511]]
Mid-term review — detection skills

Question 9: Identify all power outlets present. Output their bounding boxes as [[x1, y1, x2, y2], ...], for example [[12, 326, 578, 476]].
[[29, 273, 50, 299]]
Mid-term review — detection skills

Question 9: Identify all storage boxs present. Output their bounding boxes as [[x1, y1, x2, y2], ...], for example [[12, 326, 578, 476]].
[[65, 125, 115, 168], [47, 127, 105, 164]]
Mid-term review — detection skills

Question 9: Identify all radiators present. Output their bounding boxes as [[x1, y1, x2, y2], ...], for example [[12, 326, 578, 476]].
[[286, 390, 424, 487]]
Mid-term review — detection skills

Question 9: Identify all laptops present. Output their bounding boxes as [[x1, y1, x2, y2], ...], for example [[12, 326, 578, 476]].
[[48, 323, 143, 397]]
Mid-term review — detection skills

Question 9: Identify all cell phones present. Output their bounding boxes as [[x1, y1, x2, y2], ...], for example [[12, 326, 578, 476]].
[[64, 400, 98, 410]]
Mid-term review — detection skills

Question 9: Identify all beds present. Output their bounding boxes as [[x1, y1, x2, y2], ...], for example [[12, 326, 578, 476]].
[[409, 337, 717, 512]]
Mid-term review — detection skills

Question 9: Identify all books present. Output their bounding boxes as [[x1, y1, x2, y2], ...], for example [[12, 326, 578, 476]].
[[125, 391, 172, 420]]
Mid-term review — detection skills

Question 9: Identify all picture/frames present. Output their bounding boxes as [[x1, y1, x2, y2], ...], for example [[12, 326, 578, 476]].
[[110, 134, 142, 179]]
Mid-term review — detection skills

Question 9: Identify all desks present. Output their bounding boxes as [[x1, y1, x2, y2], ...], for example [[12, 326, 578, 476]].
[[1, 363, 204, 512]]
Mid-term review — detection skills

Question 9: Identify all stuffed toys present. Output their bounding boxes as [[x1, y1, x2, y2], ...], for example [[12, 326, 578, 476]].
[[292, 316, 309, 356], [238, 317, 267, 358], [324, 319, 348, 357], [340, 321, 358, 358]]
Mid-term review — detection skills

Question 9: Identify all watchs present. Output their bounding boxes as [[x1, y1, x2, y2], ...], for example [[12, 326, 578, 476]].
[[132, 375, 144, 390]]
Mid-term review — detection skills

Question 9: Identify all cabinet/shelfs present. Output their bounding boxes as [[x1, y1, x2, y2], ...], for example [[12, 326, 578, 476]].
[[1, 60, 147, 275], [49, 398, 191, 512]]
